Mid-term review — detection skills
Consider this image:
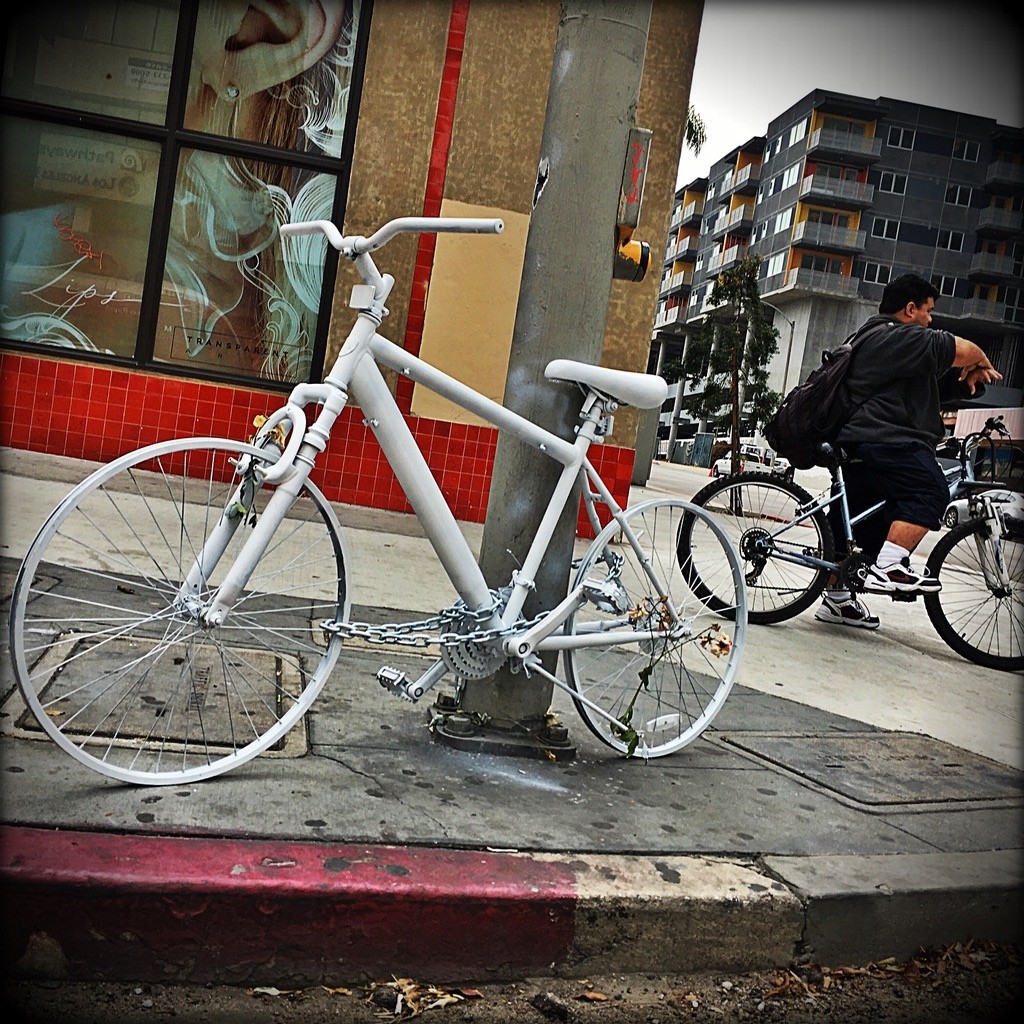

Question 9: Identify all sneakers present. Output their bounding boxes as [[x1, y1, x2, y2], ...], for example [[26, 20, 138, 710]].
[[814, 593, 880, 628], [863, 557, 942, 592]]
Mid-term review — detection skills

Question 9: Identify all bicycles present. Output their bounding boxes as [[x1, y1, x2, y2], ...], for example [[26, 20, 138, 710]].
[[7, 216, 751, 789], [674, 414, 1024, 673]]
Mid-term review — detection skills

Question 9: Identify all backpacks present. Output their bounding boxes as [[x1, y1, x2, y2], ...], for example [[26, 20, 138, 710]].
[[761, 321, 900, 468]]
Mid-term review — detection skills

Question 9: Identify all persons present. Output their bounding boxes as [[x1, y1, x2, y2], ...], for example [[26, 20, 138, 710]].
[[0, 1, 346, 315], [815, 273, 1003, 629]]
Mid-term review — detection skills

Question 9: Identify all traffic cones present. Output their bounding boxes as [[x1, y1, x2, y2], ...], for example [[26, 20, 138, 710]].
[[708, 468, 712, 477]]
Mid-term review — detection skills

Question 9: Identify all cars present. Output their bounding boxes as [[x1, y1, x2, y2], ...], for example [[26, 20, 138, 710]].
[[944, 489, 1023, 529], [714, 446, 790, 480]]
